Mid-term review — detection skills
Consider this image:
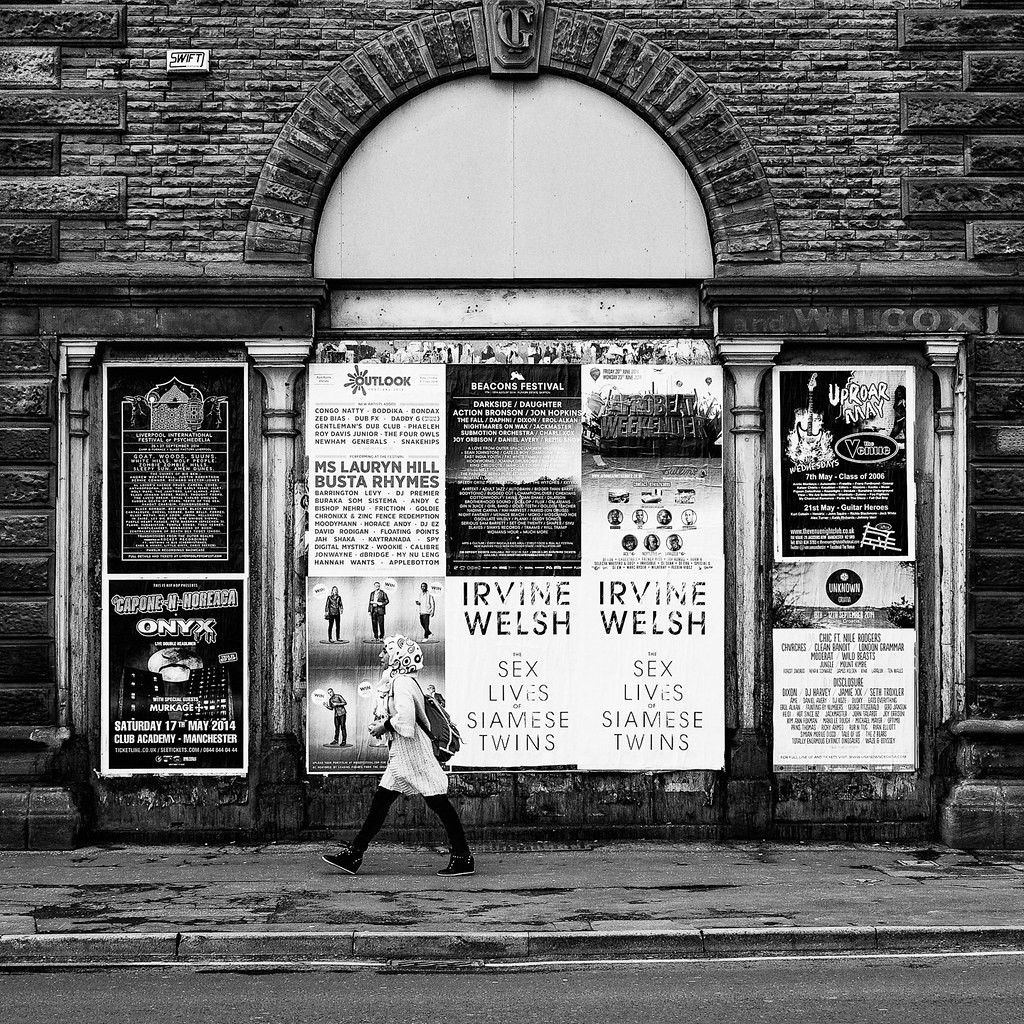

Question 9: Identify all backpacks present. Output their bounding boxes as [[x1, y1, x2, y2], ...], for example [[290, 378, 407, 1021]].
[[411, 676, 460, 762]]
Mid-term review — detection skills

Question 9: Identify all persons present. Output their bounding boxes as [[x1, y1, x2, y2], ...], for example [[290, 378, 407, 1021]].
[[368, 582, 390, 643], [417, 582, 434, 643], [321, 634, 476, 877], [323, 688, 347, 746], [426, 684, 445, 709], [324, 586, 344, 643]]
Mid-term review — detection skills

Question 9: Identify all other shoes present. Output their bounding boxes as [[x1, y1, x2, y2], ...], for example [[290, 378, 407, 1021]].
[[436, 852, 475, 876], [321, 845, 363, 874]]
